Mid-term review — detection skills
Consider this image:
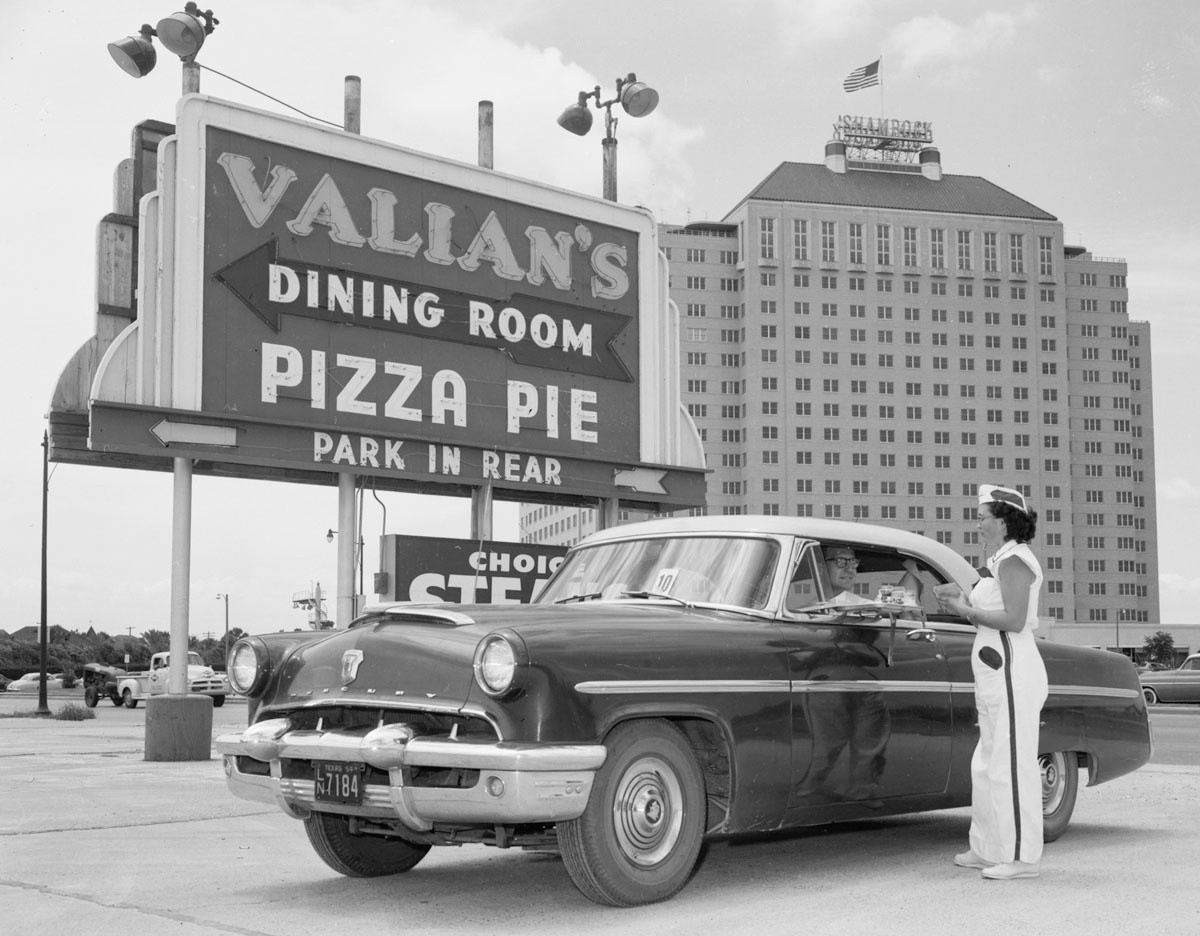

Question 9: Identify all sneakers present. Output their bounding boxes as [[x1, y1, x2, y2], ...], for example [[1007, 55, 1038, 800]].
[[954, 851, 987, 869], [981, 859, 1040, 879]]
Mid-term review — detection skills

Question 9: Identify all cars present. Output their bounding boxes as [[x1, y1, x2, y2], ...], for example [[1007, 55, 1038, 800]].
[[214, 514, 1159, 909], [1138, 653, 1200, 704], [6, 672, 64, 694]]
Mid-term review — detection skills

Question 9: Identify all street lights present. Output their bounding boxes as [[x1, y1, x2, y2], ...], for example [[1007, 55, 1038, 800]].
[[1115, 608, 1126, 653], [557, 70, 660, 532], [326, 528, 365, 595], [216, 592, 229, 672], [106, 1, 218, 693]]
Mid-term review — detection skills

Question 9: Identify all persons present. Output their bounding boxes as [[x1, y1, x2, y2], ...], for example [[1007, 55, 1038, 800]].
[[822, 546, 859, 597], [937, 484, 1050, 878], [769, 627, 893, 808]]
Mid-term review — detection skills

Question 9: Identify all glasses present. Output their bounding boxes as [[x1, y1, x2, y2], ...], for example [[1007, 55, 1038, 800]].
[[824, 557, 861, 569], [977, 515, 999, 522]]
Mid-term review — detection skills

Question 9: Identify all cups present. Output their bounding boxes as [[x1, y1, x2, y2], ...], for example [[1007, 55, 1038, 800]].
[[878, 584, 916, 606]]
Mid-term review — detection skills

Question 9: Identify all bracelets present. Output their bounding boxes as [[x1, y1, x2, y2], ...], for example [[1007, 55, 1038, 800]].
[[966, 607, 973, 621]]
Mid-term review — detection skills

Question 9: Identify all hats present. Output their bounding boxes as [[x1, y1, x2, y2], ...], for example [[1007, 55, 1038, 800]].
[[978, 484, 1028, 516]]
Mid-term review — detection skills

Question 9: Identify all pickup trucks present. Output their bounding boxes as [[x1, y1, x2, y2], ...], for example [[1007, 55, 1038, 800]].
[[118, 650, 234, 709]]
